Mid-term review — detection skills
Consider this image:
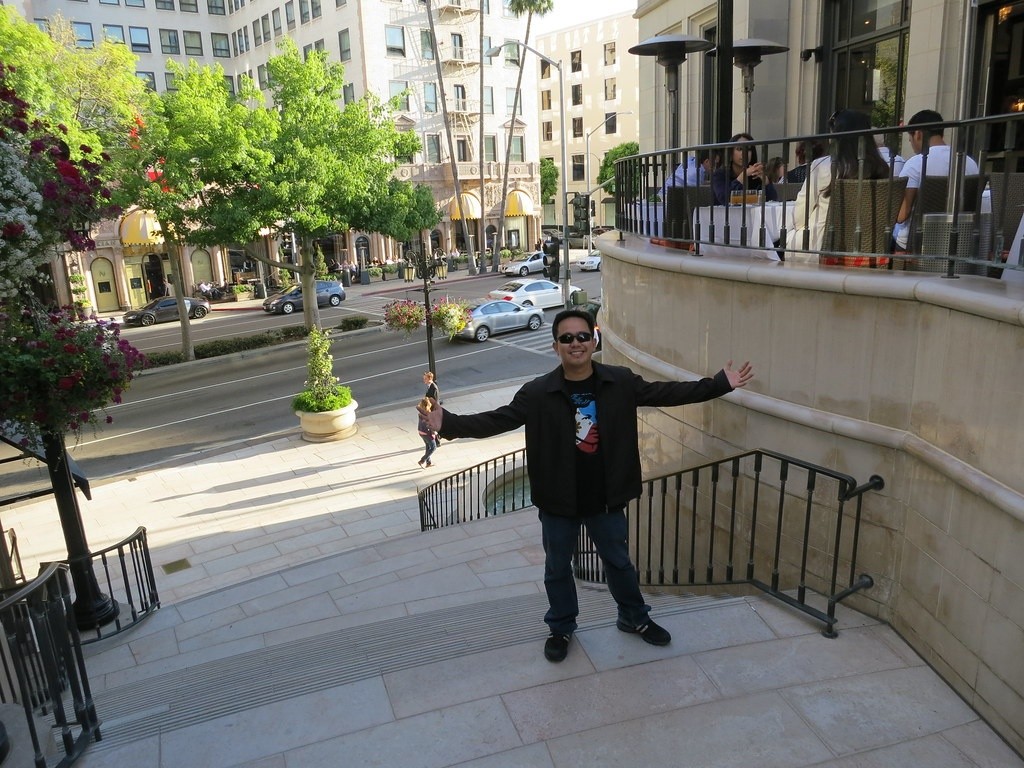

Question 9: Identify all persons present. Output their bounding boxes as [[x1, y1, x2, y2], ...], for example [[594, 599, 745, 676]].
[[330, 259, 356, 275], [243, 258, 253, 272], [417, 397, 436, 468], [658, 110, 980, 261], [199, 281, 222, 301], [537, 238, 541, 244], [162, 278, 172, 297], [223, 278, 230, 293], [372, 256, 403, 266], [448, 248, 459, 258], [423, 372, 442, 447], [416, 310, 755, 661]]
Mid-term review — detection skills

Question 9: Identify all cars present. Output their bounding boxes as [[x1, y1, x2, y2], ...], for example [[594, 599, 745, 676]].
[[262, 279, 347, 315], [542, 228, 564, 241], [576, 249, 601, 272], [452, 298, 545, 342], [485, 278, 583, 310], [499, 251, 549, 277], [122, 296, 211, 327]]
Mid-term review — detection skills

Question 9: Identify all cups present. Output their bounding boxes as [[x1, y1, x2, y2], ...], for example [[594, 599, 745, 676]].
[[745, 190, 757, 204], [730, 190, 742, 206]]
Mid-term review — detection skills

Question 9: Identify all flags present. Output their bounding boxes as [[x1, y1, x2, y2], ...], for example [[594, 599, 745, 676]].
[[130, 116, 170, 193]]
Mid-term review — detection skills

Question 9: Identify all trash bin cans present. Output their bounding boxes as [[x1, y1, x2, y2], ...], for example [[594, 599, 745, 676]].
[[254, 284, 266, 299], [448, 259, 458, 272]]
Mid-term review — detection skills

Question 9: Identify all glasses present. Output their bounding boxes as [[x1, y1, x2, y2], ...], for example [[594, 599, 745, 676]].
[[556, 331, 593, 344], [829, 112, 836, 120]]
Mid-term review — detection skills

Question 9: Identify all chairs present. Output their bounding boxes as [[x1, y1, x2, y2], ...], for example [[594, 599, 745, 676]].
[[667, 185, 710, 241], [910, 173, 990, 256], [774, 183, 803, 202], [988, 171, 1024, 261], [818, 177, 910, 267]]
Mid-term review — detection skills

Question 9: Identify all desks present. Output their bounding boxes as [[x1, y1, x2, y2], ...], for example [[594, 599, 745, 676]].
[[629, 201, 667, 239], [693, 201, 794, 262]]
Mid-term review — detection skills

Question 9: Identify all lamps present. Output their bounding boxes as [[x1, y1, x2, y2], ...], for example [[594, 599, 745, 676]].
[[800, 45, 824, 63]]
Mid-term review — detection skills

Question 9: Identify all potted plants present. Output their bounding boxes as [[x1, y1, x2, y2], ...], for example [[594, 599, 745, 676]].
[[453, 255, 468, 271], [382, 264, 399, 281], [477, 252, 493, 266], [367, 267, 383, 283], [232, 285, 255, 302], [513, 249, 525, 261], [500, 249, 512, 264]]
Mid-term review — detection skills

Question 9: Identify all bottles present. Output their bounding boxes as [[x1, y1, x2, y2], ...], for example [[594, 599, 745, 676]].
[[758, 190, 766, 204]]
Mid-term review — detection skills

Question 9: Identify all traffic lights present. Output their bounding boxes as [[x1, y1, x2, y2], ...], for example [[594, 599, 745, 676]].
[[571, 197, 590, 235], [542, 243, 559, 283]]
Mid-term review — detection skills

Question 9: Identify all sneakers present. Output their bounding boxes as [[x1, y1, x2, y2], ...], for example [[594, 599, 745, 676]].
[[544, 631, 572, 663], [616, 619, 671, 646]]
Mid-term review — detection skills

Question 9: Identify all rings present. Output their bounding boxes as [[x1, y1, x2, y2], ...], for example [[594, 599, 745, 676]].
[[740, 378, 742, 382]]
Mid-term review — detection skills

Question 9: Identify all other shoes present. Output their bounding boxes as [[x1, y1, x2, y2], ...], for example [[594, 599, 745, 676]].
[[418, 461, 425, 470], [427, 464, 435, 467]]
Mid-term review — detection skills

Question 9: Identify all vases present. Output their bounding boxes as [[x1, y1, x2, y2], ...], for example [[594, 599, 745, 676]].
[[294, 397, 359, 443]]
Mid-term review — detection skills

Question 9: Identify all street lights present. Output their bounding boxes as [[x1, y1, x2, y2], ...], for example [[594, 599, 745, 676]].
[[485, 39, 572, 309], [586, 111, 633, 194]]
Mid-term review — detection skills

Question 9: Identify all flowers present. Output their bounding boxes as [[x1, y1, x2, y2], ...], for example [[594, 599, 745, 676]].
[[0, 297, 152, 476], [382, 299, 425, 343], [302, 323, 341, 400], [424, 293, 473, 345], [0, 62, 125, 299]]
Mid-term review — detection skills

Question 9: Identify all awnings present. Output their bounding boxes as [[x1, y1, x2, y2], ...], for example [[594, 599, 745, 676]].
[[450, 193, 482, 220], [119, 208, 175, 246], [505, 190, 534, 216]]
